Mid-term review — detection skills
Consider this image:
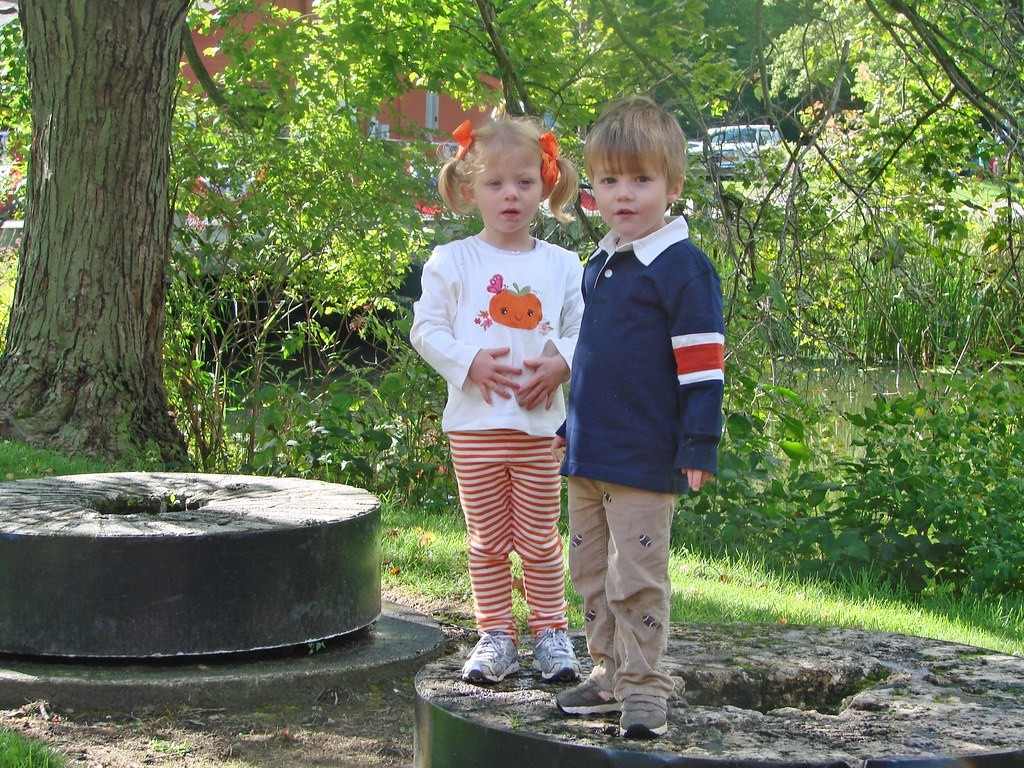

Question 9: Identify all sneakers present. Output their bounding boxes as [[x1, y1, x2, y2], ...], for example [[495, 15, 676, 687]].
[[530, 628, 582, 683], [461, 631, 520, 685], [619, 679, 668, 741], [557, 670, 622, 713]]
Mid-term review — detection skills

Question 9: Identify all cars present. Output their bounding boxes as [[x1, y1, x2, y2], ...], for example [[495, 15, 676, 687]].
[[689, 125, 783, 181]]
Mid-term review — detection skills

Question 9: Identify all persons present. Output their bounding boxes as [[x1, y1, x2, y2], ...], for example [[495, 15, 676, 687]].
[[551, 95, 726, 739], [409, 112, 585, 684]]
